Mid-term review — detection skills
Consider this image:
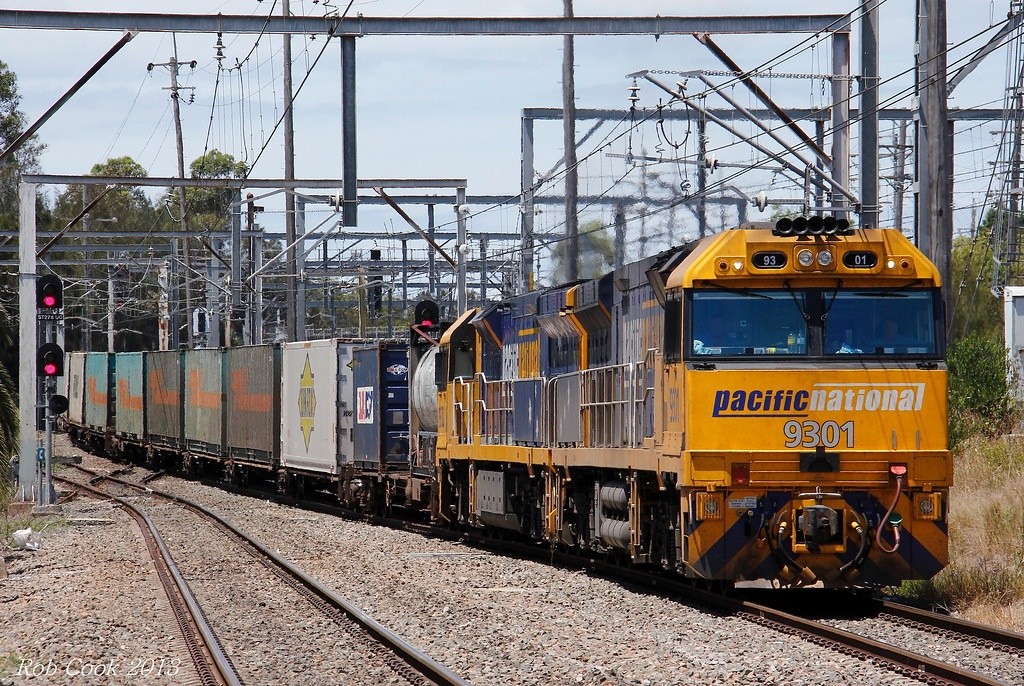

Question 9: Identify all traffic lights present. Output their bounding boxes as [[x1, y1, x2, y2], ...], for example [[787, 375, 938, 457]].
[[36, 275, 63, 309], [35, 344, 65, 377], [415, 300, 439, 324]]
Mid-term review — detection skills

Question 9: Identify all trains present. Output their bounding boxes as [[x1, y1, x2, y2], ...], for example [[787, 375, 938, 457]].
[[70, 214, 955, 595]]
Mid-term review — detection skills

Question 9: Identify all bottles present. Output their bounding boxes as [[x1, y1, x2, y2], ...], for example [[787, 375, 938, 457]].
[[788, 331, 796, 353], [797, 330, 806, 354]]
